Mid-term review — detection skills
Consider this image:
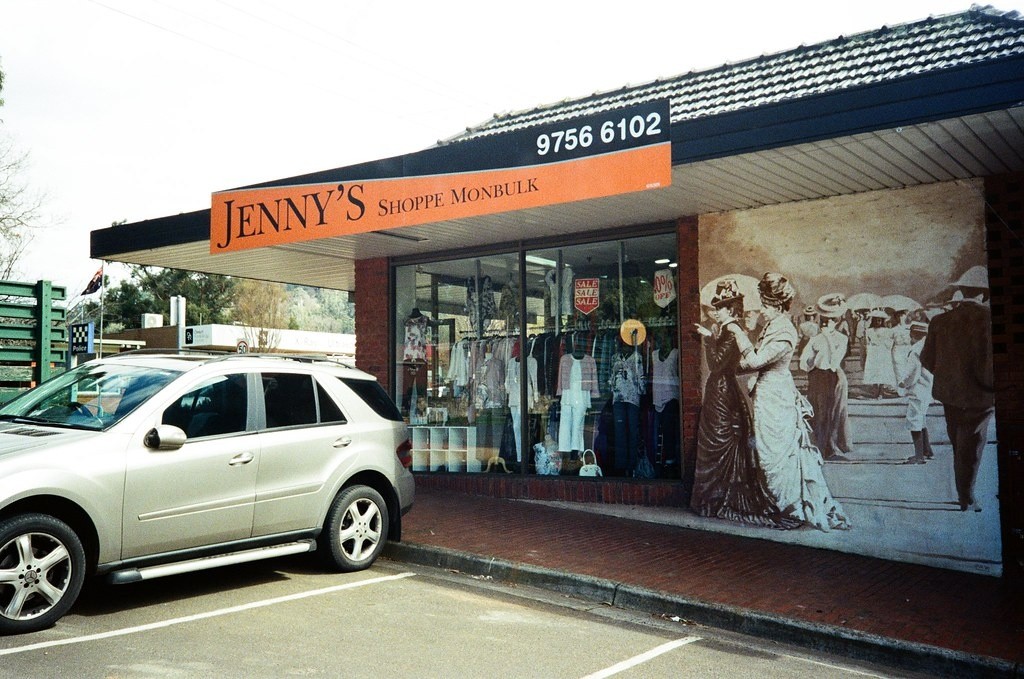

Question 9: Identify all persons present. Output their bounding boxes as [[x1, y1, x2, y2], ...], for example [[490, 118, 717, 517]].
[[555, 338, 601, 461], [399, 308, 434, 417], [798, 266, 994, 513], [693, 271, 853, 532], [475, 353, 539, 462], [608, 326, 681, 483]]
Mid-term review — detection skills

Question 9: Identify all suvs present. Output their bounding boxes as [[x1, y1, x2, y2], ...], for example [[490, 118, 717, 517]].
[[0, 348, 416, 637]]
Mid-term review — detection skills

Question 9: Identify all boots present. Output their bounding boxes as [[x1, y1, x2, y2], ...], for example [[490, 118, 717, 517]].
[[903, 431, 926, 464], [908, 428, 933, 460]]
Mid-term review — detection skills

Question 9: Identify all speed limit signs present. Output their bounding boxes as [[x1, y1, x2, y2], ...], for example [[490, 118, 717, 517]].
[[237, 341, 247, 354]]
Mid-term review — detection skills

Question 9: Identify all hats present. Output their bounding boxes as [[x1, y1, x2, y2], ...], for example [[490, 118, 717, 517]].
[[947, 290, 964, 302], [814, 293, 847, 317], [804, 306, 816, 315], [905, 322, 928, 332], [757, 272, 794, 305], [949, 266, 989, 288], [711, 278, 744, 306], [866, 310, 888, 318]]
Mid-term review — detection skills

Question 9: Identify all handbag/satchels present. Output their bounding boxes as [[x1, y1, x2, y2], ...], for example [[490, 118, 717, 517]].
[[633, 446, 656, 479], [579, 449, 603, 476]]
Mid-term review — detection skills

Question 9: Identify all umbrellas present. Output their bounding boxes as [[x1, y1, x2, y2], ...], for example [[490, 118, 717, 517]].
[[882, 295, 924, 313], [846, 293, 889, 313], [700, 274, 763, 312]]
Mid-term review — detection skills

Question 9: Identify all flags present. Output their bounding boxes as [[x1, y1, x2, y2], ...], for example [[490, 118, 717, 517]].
[[81, 265, 102, 296]]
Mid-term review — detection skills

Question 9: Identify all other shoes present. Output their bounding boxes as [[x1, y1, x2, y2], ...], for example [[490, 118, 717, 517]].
[[960, 503, 982, 512]]
